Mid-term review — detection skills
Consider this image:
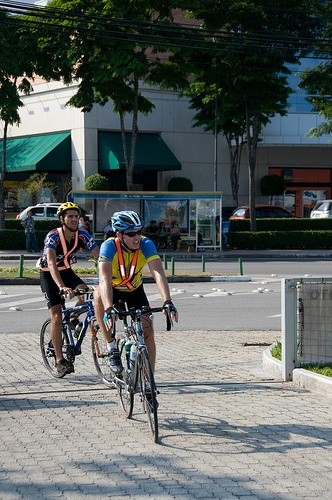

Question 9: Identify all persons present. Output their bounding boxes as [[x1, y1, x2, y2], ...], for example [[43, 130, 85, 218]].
[[78, 210, 91, 254], [103, 220, 113, 241], [36, 202, 99, 375], [141, 220, 180, 251], [21, 211, 41, 253], [94, 211, 178, 412]]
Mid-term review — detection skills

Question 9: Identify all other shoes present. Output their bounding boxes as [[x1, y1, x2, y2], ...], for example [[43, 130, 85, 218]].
[[57, 358, 74, 374], [27, 249, 42, 253], [69, 318, 82, 329]]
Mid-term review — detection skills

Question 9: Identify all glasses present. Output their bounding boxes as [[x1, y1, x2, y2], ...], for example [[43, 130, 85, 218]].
[[123, 230, 142, 237]]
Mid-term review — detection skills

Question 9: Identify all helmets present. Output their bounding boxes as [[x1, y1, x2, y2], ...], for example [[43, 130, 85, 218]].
[[110, 210, 143, 234], [57, 202, 81, 220]]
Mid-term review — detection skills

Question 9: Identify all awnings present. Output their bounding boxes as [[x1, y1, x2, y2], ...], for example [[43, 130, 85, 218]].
[[98, 130, 182, 172], [0, 133, 72, 171]]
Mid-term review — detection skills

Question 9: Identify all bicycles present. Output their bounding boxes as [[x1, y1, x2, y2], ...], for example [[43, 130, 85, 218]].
[[39, 287, 120, 389], [99, 298, 175, 445]]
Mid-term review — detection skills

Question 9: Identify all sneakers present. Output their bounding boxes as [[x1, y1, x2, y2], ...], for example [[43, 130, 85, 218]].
[[142, 384, 160, 408], [105, 339, 124, 374]]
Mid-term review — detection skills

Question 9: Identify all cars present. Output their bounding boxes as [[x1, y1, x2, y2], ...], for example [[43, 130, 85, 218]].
[[229, 206, 300, 218]]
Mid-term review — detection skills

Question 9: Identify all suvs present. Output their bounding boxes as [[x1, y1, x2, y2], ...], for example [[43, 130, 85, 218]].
[[19, 201, 90, 225], [309, 199, 332, 219]]
